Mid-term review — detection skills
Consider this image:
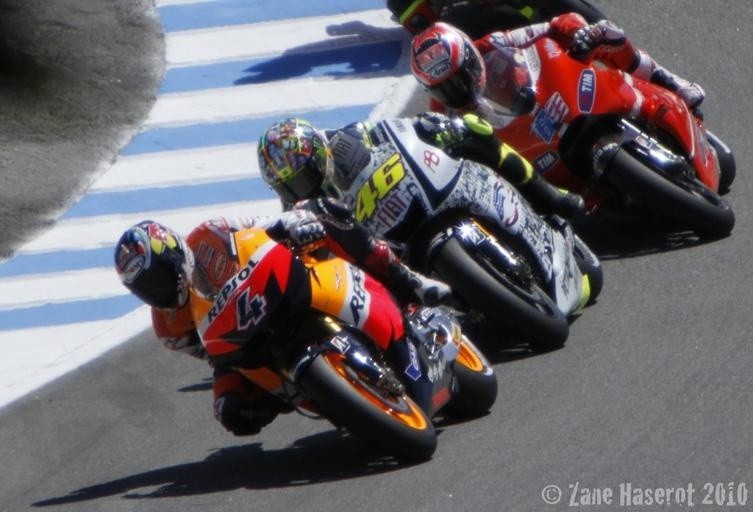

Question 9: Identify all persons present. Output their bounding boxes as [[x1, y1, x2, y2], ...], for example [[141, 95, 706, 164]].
[[115, 1, 705, 474]]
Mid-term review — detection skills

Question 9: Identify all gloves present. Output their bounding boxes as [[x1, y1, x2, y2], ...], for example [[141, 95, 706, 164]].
[[413, 114, 466, 151], [573, 24, 604, 54], [279, 213, 325, 242]]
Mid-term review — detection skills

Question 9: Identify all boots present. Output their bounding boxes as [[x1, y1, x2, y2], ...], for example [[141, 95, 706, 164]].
[[521, 169, 585, 218], [380, 259, 453, 307], [651, 57, 703, 107]]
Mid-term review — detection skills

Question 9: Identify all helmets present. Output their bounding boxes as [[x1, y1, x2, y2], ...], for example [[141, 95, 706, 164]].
[[257, 119, 335, 206], [113, 221, 193, 312], [410, 21, 486, 110]]
